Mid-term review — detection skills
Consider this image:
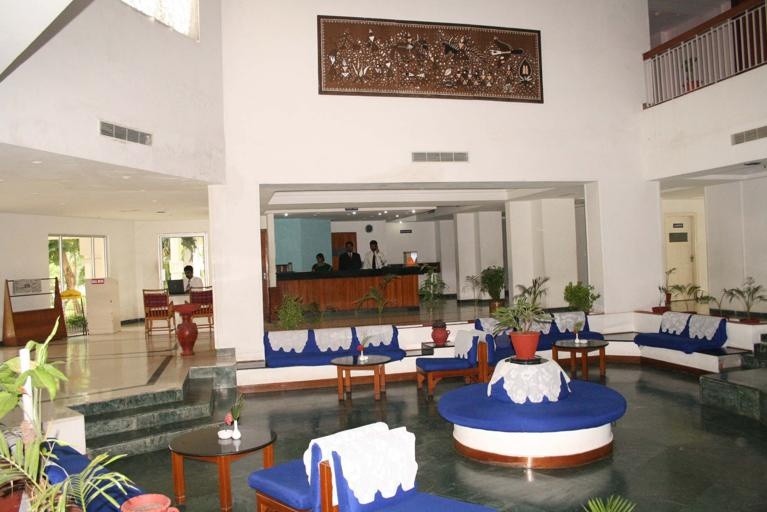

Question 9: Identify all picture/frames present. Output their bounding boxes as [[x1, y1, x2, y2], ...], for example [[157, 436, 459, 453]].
[[317, 15, 544, 104]]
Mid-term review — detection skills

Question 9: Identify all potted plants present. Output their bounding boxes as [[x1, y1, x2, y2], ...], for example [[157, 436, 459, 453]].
[[650, 285, 670, 314], [725, 276, 766, 325], [481, 264, 508, 313], [671, 283, 700, 315], [492, 298, 558, 360]]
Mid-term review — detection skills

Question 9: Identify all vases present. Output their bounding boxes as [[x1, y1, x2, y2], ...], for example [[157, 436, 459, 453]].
[[359, 351, 365, 360], [232, 419, 240, 439]]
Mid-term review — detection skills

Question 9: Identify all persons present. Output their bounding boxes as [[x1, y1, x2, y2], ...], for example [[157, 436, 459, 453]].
[[339, 242, 361, 270], [183, 266, 203, 292], [360, 240, 386, 269], [312, 253, 333, 272]]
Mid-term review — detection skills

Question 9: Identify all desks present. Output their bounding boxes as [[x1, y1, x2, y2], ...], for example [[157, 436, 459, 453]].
[[167, 423, 278, 512], [552, 340, 610, 380], [331, 354, 387, 400]]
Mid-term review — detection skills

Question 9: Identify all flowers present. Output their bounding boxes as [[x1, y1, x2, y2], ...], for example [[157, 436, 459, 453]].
[[357, 336, 371, 351], [222, 395, 248, 425]]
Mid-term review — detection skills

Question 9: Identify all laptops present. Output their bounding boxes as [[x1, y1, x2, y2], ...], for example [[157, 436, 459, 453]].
[[166, 280, 185, 295]]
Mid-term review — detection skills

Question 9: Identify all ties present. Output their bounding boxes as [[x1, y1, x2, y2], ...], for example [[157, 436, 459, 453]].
[[349, 252, 352, 261], [371, 251, 376, 269], [186, 280, 190, 290]]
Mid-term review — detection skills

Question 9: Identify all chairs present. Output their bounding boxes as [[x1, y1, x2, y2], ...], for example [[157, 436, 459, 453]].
[[142, 289, 177, 341], [188, 286, 213, 332], [245, 422, 389, 511], [416, 330, 483, 400], [320, 426, 500, 512]]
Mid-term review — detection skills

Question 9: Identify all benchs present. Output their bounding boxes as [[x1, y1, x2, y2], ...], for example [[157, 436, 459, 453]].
[[437, 379, 628, 471], [635, 312, 752, 374], [266, 325, 413, 389], [476, 311, 604, 372]]
